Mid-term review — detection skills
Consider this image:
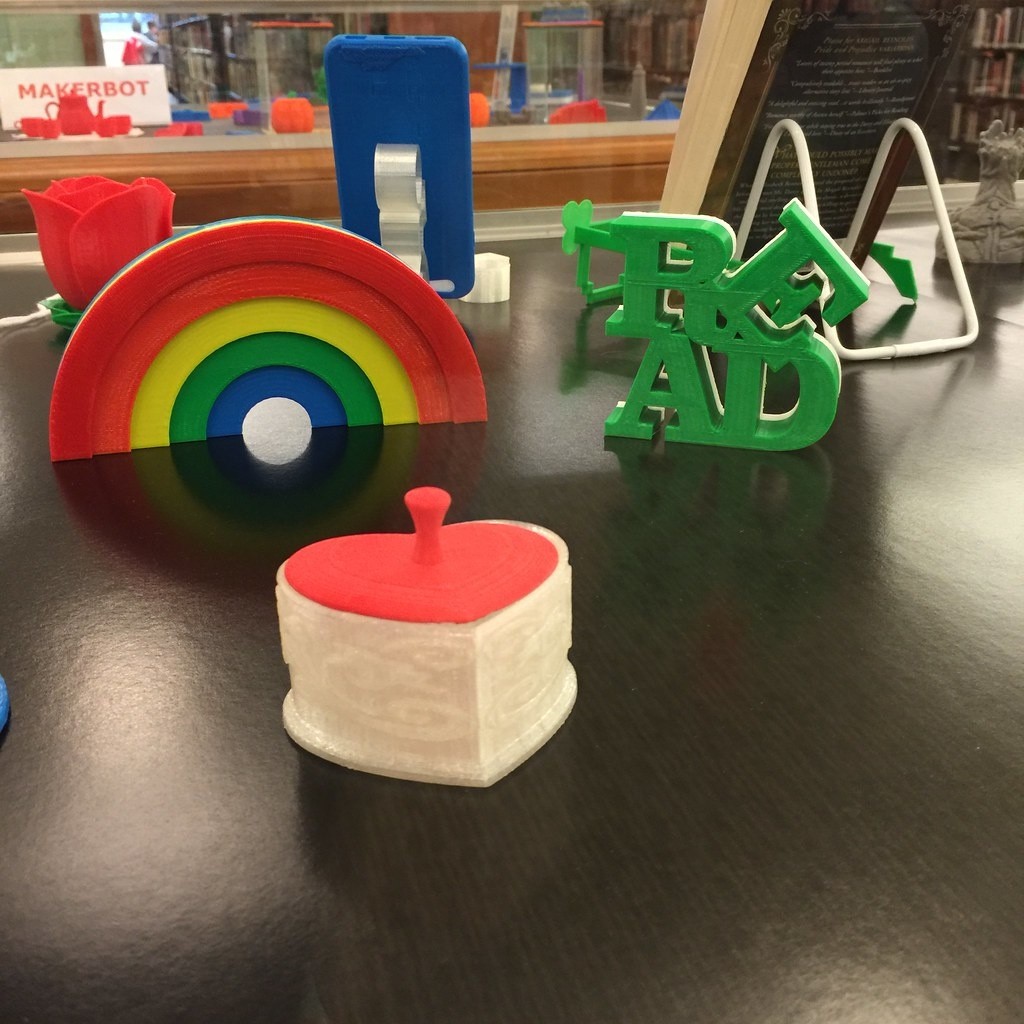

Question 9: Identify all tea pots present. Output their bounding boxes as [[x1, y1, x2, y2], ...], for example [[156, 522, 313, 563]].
[[45, 94, 103, 135]]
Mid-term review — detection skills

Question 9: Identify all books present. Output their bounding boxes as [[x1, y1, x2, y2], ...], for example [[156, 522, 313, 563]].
[[965, 50, 1024, 100], [971, 5, 1024, 49]]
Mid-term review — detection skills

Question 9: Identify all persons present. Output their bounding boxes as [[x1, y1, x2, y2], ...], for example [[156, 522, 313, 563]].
[[121, 20, 159, 64], [948, 103, 1024, 144]]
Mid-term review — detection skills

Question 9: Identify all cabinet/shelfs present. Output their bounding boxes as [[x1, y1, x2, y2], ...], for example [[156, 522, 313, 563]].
[[890, 0, 1024, 189]]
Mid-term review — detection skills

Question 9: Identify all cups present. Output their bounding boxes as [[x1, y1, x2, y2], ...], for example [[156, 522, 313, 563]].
[[38, 121, 60, 138], [14, 118, 41, 136], [95, 118, 115, 136], [110, 115, 130, 134]]
